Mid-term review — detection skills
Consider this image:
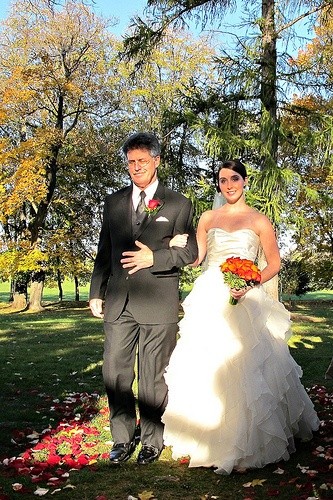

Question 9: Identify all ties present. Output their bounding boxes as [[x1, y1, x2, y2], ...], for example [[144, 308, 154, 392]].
[[136, 190, 146, 218]]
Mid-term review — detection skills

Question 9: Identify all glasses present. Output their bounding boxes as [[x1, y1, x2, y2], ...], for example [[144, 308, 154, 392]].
[[126, 157, 153, 168]]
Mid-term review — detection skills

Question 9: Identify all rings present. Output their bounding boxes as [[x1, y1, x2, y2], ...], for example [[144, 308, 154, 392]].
[[130, 258, 133, 262]]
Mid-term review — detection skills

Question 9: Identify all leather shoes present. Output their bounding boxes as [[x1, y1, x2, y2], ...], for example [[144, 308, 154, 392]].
[[137, 444, 159, 464], [110, 435, 136, 463]]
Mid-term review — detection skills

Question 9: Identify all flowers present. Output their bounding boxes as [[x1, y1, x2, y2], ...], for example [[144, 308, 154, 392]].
[[218, 255, 262, 305], [143, 200, 159, 223]]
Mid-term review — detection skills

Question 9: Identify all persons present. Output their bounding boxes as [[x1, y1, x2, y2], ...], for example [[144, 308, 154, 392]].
[[161, 161, 320, 478], [87, 132, 198, 464]]
